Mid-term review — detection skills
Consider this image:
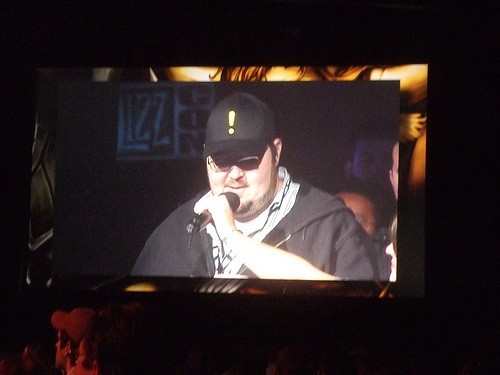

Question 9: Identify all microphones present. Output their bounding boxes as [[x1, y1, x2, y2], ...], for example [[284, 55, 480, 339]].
[[186, 192, 240, 234]]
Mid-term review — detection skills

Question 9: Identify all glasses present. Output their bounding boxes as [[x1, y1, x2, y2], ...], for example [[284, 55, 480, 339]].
[[207, 145, 270, 172]]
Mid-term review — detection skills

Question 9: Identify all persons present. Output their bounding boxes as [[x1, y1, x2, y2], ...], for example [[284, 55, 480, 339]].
[[323, 175, 380, 240], [99, 297, 500, 375], [130, 92, 378, 280], [0, 325, 51, 374], [327, 128, 393, 226], [385, 213, 397, 283], [51, 306, 104, 375], [388, 142, 397, 207]]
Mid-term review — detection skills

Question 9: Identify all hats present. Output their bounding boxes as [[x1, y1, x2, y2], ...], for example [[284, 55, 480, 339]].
[[203, 92, 276, 157], [51, 308, 98, 343]]
